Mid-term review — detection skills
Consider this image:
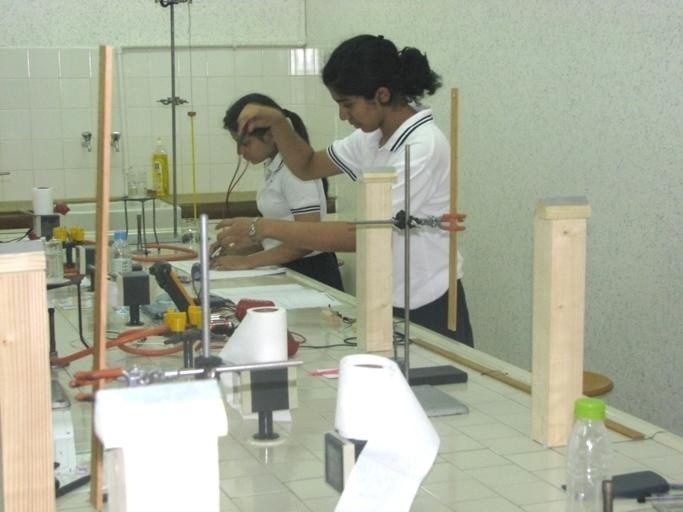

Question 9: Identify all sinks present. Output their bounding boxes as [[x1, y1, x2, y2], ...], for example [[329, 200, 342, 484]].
[[54, 199, 181, 232]]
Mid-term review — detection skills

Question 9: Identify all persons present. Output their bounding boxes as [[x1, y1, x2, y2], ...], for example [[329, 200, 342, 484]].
[[209, 92, 344, 295], [215, 34, 474, 351]]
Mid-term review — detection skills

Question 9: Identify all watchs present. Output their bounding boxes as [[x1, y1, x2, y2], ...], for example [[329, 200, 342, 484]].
[[247, 217, 260, 243]]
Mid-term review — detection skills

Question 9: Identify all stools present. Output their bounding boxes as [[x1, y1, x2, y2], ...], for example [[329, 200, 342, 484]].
[[582, 370, 613, 397]]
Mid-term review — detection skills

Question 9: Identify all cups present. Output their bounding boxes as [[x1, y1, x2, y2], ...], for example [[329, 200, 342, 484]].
[[126, 173, 147, 199]]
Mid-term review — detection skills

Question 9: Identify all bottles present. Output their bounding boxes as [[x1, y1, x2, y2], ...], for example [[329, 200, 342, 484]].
[[566, 397, 611, 511], [109, 229, 131, 316]]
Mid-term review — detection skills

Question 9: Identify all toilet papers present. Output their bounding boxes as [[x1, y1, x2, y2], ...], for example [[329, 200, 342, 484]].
[[333, 354, 440, 512], [32, 186, 53, 215], [219, 306, 290, 423]]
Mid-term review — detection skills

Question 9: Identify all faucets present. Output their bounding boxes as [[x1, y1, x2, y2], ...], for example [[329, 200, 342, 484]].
[[81, 131, 93, 151], [111, 132, 120, 153]]
